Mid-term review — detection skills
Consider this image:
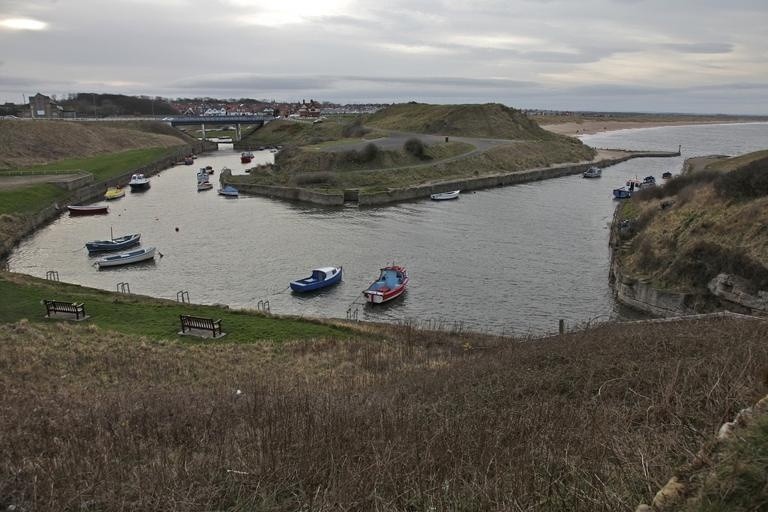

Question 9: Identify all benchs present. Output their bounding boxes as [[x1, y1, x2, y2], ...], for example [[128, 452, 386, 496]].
[[43, 300, 85, 319], [180, 315, 221, 337]]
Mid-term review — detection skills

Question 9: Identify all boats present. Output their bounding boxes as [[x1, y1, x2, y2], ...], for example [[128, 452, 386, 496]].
[[431, 188, 461, 200], [183, 152, 215, 191], [363, 260, 409, 304], [103, 186, 125, 200], [218, 185, 239, 196], [92, 246, 155, 269], [86, 228, 138, 253], [128, 173, 149, 189], [289, 266, 342, 294], [614, 168, 672, 200], [67, 205, 109, 215], [239, 144, 283, 164], [584, 166, 601, 177]]
